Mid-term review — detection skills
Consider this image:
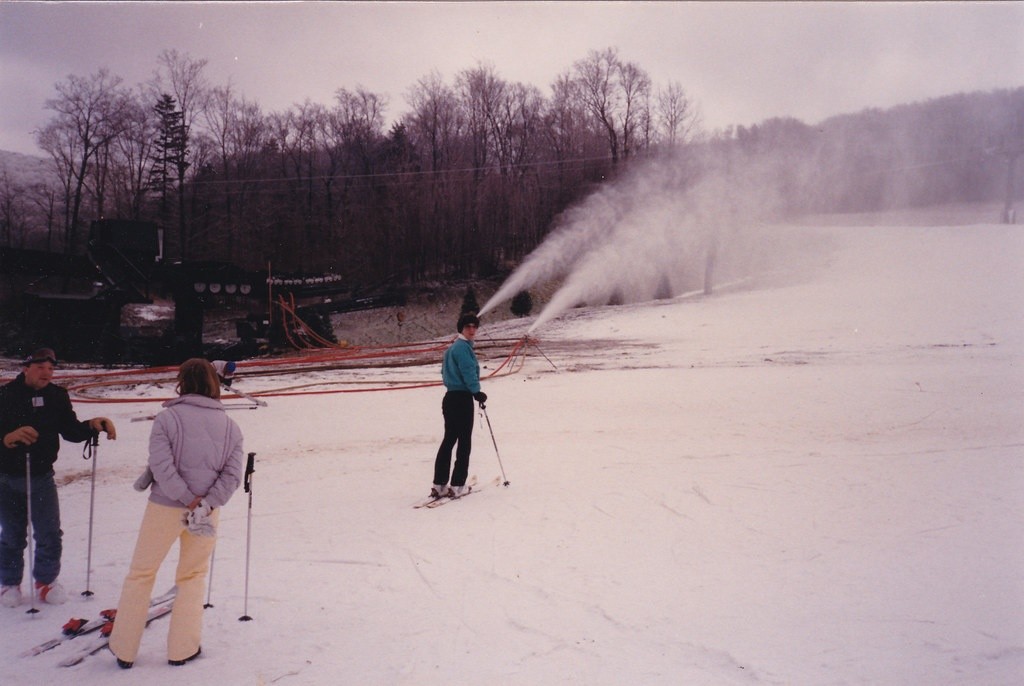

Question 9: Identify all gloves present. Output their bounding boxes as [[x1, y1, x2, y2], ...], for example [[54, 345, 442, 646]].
[[474, 392, 487, 403], [89, 417, 116, 440], [182, 499, 216, 537], [4, 426, 39, 449]]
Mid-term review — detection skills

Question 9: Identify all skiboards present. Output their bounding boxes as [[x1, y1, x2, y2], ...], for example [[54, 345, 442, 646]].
[[15, 583, 177, 668], [412, 474, 503, 510]]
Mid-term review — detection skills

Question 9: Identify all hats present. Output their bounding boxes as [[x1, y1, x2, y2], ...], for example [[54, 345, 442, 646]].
[[226, 362, 236, 373]]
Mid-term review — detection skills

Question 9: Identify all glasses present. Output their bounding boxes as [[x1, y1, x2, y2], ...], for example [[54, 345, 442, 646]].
[[464, 325, 477, 331], [30, 348, 56, 363]]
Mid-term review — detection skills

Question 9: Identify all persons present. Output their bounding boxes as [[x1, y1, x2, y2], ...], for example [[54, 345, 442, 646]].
[[210, 360, 236, 391], [0, 348, 116, 608], [430, 313, 488, 499], [107, 358, 243, 669]]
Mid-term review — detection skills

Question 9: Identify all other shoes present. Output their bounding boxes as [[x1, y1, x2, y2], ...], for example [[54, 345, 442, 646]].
[[169, 647, 201, 666], [117, 658, 133, 668], [35, 584, 63, 603], [450, 486, 472, 497], [432, 484, 449, 496], [0, 585, 21, 606]]
